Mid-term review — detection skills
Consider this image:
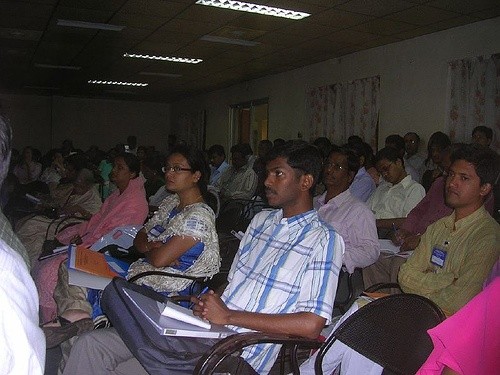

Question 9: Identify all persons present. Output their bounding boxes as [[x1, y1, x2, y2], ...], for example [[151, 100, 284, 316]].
[[1, 122, 500, 375]]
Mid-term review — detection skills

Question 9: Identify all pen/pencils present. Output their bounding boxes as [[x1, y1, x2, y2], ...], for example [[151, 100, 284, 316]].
[[392, 219, 403, 246], [188, 287, 209, 309]]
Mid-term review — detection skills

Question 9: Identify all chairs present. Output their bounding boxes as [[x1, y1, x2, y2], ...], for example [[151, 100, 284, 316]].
[[195, 292, 447, 375]]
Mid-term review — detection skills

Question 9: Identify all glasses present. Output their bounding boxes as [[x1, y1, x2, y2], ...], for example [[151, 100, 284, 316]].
[[404, 139, 418, 144], [161, 165, 194, 173], [322, 160, 352, 172], [377, 160, 394, 176]]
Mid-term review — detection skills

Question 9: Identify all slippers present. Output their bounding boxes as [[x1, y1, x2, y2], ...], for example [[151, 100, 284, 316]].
[[40, 315, 94, 349]]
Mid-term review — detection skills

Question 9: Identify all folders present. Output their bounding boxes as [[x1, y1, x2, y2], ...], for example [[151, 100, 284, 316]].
[[122, 286, 237, 338], [68, 243, 130, 280], [13, 193, 40, 213]]
